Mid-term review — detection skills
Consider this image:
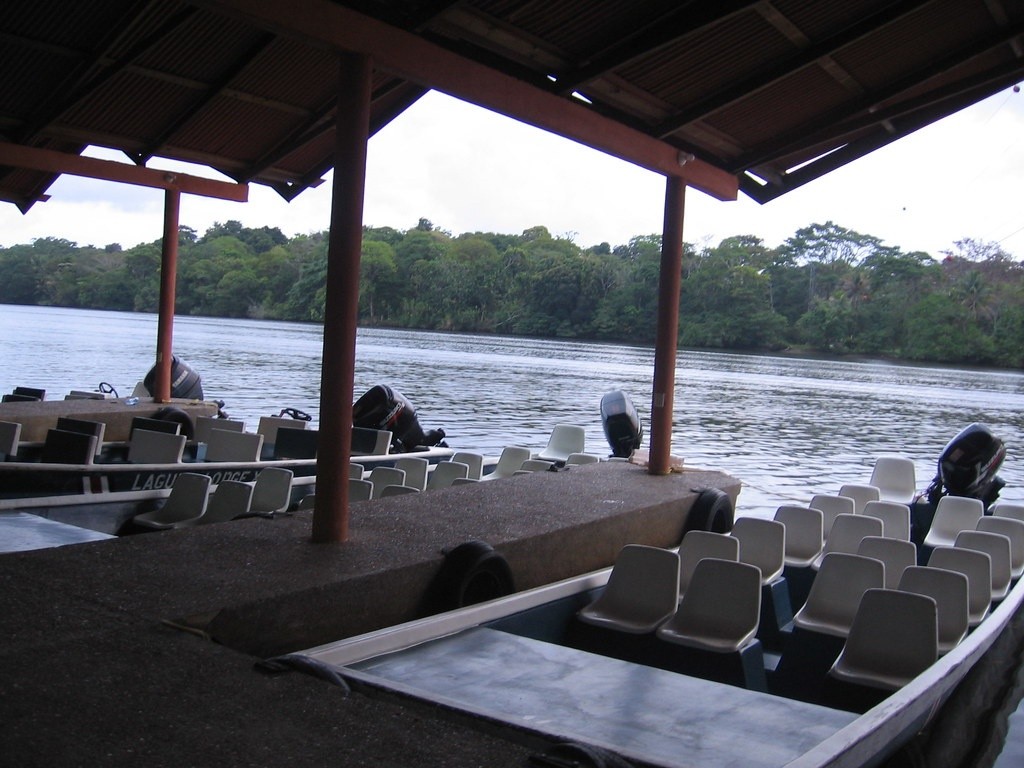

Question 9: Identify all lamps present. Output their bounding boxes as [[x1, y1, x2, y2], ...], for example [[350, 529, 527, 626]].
[[678, 150, 696, 166]]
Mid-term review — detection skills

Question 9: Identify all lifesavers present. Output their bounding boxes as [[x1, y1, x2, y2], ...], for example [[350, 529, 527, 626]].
[[431, 541, 518, 610], [154, 408, 199, 463]]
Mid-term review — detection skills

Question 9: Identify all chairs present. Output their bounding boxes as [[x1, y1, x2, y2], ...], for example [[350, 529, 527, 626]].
[[810, 512, 885, 571], [857, 536, 917, 589], [576, 544, 682, 636], [975, 515, 1024, 579], [773, 505, 824, 568], [792, 551, 886, 638], [824, 587, 939, 691], [810, 494, 855, 539], [895, 565, 970, 655], [656, 557, 762, 654], [862, 501, 911, 542], [953, 530, 1012, 602], [926, 546, 992, 628], [0, 416, 599, 528], [838, 456, 985, 548]]
